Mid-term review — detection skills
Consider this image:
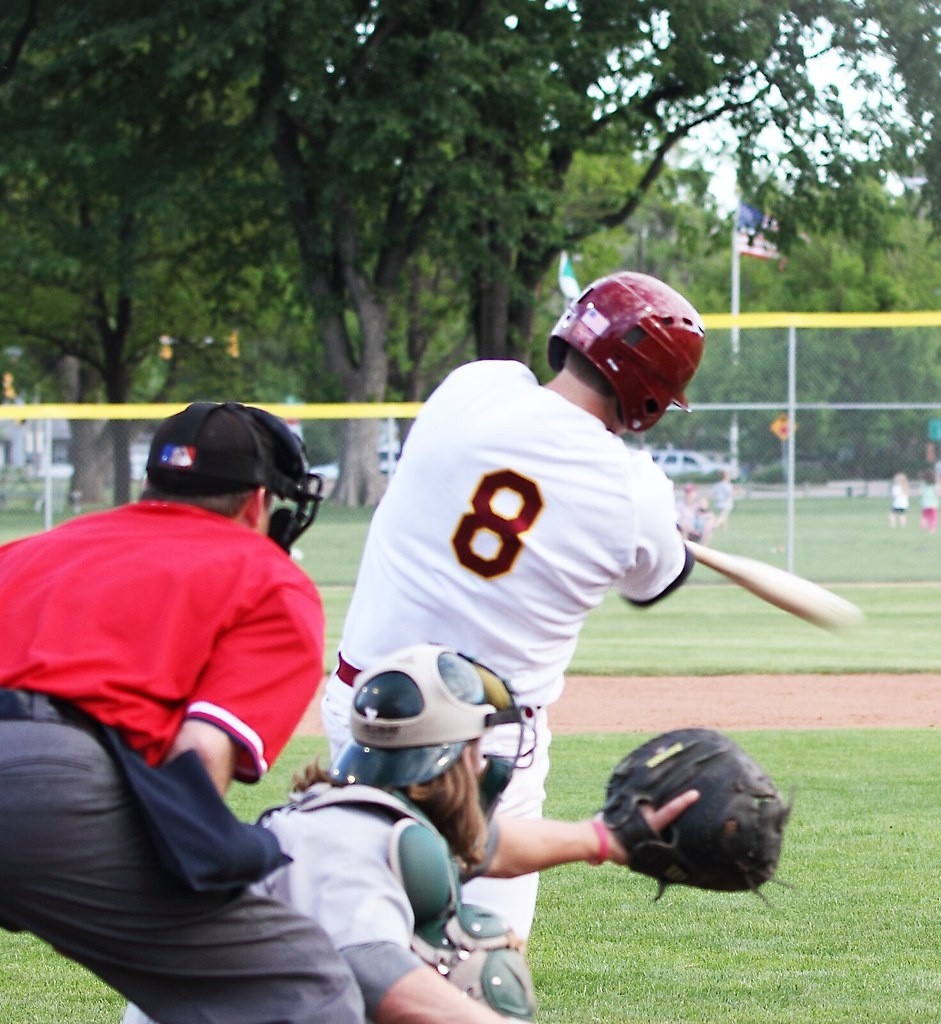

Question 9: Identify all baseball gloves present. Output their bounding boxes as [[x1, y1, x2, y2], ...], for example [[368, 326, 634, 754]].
[[606, 729, 803, 913]]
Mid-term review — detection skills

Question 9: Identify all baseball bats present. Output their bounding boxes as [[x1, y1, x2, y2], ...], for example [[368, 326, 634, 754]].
[[684, 535, 860, 635]]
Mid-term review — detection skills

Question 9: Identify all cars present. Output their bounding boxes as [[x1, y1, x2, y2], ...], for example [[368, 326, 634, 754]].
[[651, 451, 719, 475], [287, 416, 405, 480]]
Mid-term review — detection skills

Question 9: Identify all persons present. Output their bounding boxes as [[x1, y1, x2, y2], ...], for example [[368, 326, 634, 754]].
[[324, 268, 694, 949], [670, 469, 733, 545], [918, 471, 940, 531], [120, 640, 699, 1024], [890, 472, 909, 527], [0, 402, 368, 1024]]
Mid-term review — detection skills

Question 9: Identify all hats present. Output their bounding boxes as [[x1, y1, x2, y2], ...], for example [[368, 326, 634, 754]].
[[147, 401, 291, 496]]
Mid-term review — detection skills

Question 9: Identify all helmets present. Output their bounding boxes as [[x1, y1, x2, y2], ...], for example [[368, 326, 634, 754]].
[[332, 643, 498, 788], [552, 271, 706, 431]]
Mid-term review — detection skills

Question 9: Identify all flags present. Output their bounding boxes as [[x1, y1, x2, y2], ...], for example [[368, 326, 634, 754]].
[[558, 256, 581, 299], [735, 203, 809, 264]]
